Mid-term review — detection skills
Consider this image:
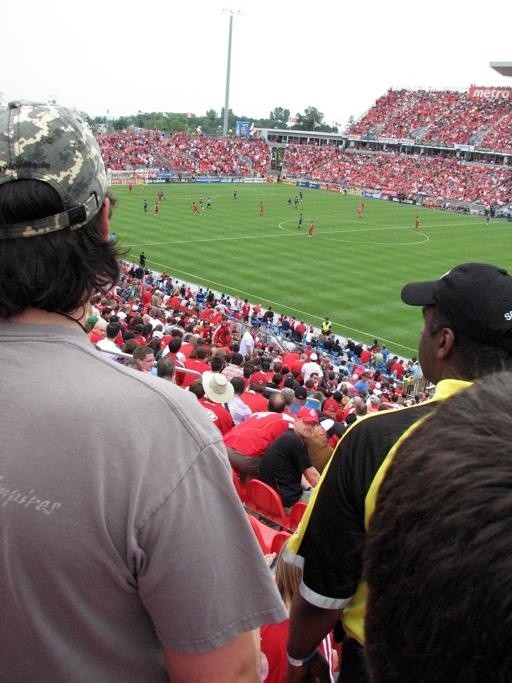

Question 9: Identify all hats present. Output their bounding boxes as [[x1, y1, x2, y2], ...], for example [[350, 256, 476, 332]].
[[295, 406, 320, 425], [201, 370, 236, 404], [309, 352, 319, 361], [0, 99, 109, 240], [401, 262, 511, 346]]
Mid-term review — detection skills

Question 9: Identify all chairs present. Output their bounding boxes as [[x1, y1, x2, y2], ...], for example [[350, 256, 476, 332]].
[[230, 466, 335, 683]]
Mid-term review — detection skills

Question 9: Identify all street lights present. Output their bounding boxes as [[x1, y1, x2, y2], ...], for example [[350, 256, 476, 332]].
[[221, 8, 243, 136]]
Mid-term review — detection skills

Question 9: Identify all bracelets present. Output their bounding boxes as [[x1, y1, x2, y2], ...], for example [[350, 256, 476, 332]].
[[287, 648, 318, 667]]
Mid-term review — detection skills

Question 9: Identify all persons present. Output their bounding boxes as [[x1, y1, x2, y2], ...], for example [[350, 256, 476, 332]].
[[143, 192, 166, 214], [283, 85, 512, 218], [297, 213, 314, 237], [128, 181, 132, 191], [95, 126, 273, 186], [415, 217, 420, 230], [363, 373, 511, 680], [0, 103, 287, 683], [192, 195, 211, 215], [86, 262, 431, 511], [259, 202, 264, 216], [139, 251, 146, 268], [486, 213, 490, 225], [234, 190, 238, 199], [278, 265, 511, 682]]
